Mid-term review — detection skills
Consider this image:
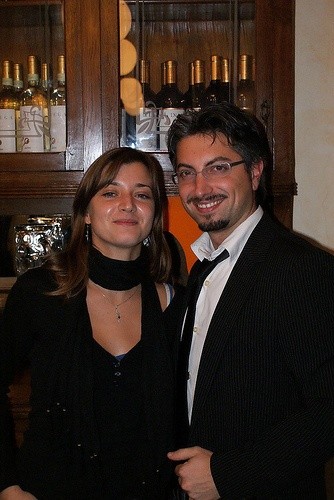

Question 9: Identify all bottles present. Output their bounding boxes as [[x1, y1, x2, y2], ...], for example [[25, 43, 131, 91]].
[[125, 53, 256, 153], [0, 54, 67, 153]]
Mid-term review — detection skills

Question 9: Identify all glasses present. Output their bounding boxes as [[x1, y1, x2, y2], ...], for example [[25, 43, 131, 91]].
[[171, 160, 245, 186]]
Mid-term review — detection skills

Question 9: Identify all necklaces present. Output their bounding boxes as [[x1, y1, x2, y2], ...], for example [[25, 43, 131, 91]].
[[94, 282, 139, 319]]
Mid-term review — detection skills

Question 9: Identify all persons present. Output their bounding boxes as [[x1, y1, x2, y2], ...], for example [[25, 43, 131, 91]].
[[165, 105, 334, 500], [0, 147, 186, 500]]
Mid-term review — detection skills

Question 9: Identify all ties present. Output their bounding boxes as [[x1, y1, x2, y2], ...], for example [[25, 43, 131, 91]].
[[175, 249, 230, 380]]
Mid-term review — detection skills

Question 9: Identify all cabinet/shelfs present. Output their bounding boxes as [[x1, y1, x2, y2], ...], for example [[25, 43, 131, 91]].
[[0, 0, 299, 233]]
